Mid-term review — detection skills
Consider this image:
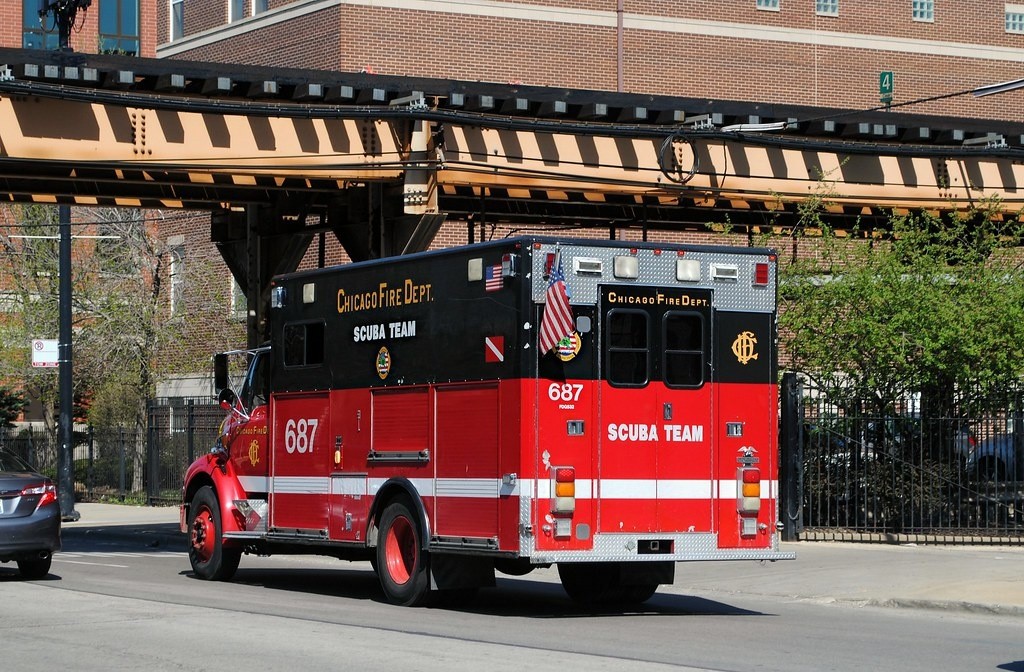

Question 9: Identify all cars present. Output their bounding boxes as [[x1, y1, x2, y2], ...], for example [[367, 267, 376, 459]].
[[855, 412, 975, 464], [0, 445, 63, 579], [969, 431, 1016, 480]]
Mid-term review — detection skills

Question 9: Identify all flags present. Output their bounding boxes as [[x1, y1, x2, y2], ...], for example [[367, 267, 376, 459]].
[[539, 252, 575, 357]]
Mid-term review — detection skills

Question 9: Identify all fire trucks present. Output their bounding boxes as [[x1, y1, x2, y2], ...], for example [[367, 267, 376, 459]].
[[179, 234, 798, 611]]
